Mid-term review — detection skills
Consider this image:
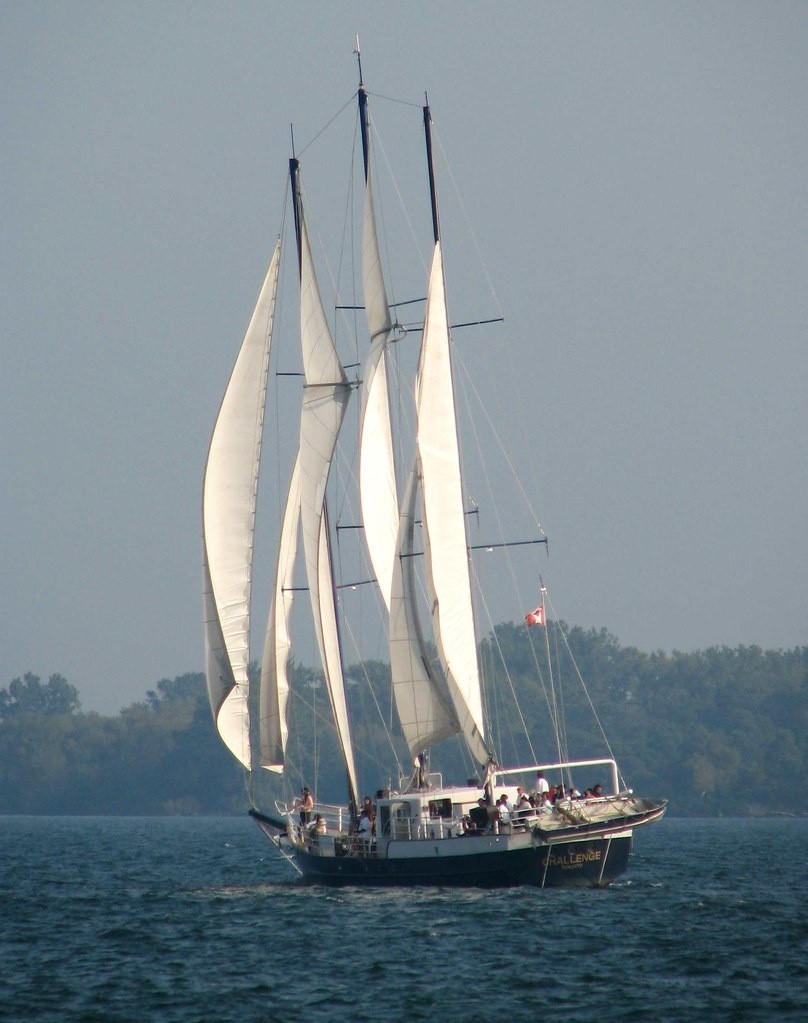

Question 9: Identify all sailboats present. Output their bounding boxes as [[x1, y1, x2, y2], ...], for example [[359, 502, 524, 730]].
[[200, 34, 669, 888]]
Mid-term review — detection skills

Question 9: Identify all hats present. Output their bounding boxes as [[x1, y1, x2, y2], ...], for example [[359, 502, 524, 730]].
[[552, 784, 559, 788], [517, 787, 522, 791], [500, 795, 508, 800], [318, 819, 326, 825], [302, 788, 309, 794]]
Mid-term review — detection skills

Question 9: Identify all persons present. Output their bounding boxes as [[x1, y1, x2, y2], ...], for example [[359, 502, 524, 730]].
[[363, 797, 376, 827], [357, 810, 371, 857], [306, 813, 327, 835], [456, 770, 602, 838], [292, 788, 313, 826]]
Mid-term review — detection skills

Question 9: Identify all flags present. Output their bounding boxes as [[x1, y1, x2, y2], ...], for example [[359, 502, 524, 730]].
[[525, 606, 544, 627]]
